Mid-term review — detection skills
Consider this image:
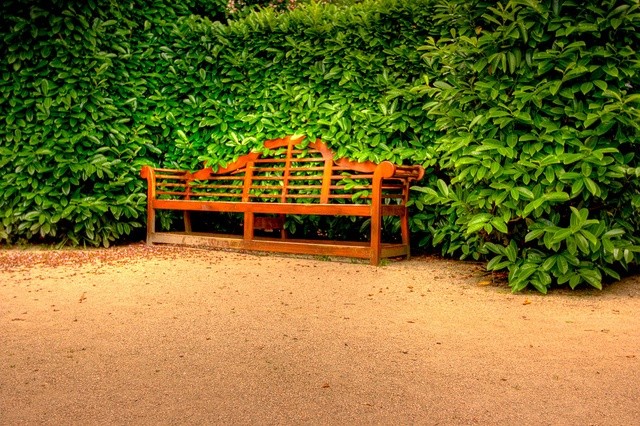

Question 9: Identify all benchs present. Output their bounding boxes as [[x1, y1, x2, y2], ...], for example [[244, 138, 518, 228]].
[[139, 134, 426, 266]]
